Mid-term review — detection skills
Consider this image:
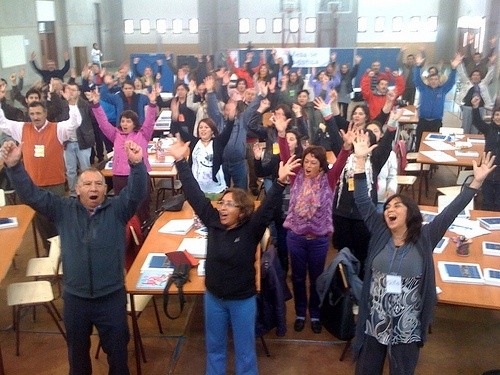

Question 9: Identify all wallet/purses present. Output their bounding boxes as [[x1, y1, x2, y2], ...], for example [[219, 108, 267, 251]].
[[165, 249, 200, 268]]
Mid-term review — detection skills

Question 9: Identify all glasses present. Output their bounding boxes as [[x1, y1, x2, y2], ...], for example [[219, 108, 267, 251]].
[[217, 201, 239, 211]]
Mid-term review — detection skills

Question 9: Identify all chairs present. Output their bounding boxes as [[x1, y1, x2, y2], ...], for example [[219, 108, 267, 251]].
[[156, 180, 182, 209], [456, 170, 475, 185], [315, 247, 363, 361], [95, 293, 165, 364], [26, 257, 63, 322], [439, 127, 464, 134], [48, 237, 60, 257], [400, 130, 437, 180], [398, 140, 430, 192], [433, 174, 474, 206], [6, 256, 67, 357], [125, 215, 145, 272], [397, 175, 418, 200]]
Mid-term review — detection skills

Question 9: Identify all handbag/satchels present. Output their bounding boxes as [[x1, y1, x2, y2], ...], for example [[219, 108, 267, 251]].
[[351, 92, 364, 102], [163, 263, 191, 320], [319, 262, 355, 341], [141, 194, 185, 230]]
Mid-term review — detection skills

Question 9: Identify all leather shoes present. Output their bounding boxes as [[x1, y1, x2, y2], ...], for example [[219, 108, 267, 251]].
[[295, 318, 306, 332], [311, 321, 321, 333]]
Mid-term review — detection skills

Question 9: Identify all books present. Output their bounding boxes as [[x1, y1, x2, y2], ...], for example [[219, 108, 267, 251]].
[[455, 150, 479, 157], [436, 194, 470, 218], [158, 219, 194, 235], [437, 261, 485, 284], [433, 236, 449, 253], [136, 270, 173, 290], [420, 210, 439, 225], [140, 252, 174, 274], [476, 217, 500, 230], [176, 237, 208, 258], [482, 241, 500, 256], [105, 161, 113, 170], [483, 267, 500, 286], [0, 217, 18, 229], [425, 133, 447, 141]]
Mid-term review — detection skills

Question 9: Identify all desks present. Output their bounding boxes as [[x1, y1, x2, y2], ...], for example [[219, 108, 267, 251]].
[[416, 131, 486, 205], [398, 105, 419, 131], [101, 141, 178, 196], [0, 204, 41, 375], [419, 204, 500, 335], [125, 201, 270, 375]]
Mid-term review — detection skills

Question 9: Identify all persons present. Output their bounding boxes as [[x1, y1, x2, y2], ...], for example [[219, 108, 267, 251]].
[[0, 29, 500, 375]]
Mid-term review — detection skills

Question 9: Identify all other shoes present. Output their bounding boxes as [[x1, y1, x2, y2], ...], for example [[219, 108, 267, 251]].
[[40, 248, 49, 257], [252, 189, 260, 196]]
